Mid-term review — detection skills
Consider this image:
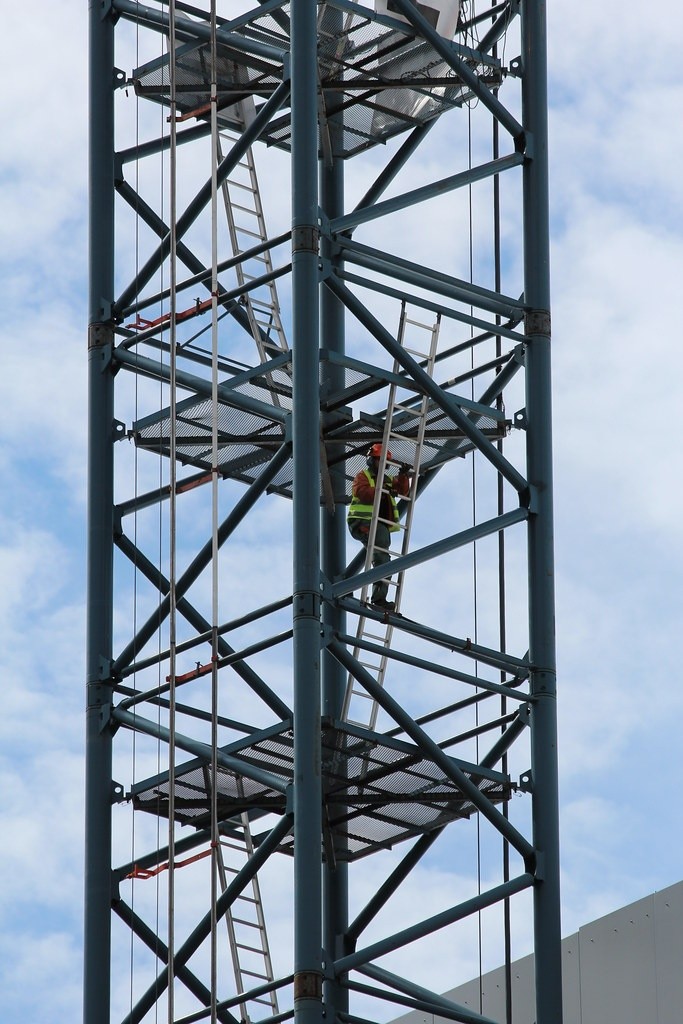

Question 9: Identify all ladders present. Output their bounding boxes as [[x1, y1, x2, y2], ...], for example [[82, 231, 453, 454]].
[[198, 758, 279, 1024], [334, 300, 442, 797], [197, 41, 294, 411]]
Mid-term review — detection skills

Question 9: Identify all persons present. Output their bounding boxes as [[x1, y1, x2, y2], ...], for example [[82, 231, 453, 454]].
[[347, 444, 413, 612]]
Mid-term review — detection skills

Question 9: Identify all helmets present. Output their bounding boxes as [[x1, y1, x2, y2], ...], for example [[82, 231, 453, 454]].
[[368, 443, 391, 459]]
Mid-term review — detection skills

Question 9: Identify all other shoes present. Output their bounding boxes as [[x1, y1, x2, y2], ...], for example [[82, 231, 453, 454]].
[[372, 600, 395, 613]]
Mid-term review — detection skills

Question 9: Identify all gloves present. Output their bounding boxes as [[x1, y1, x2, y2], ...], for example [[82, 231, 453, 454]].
[[385, 487, 399, 498], [399, 463, 413, 476]]
